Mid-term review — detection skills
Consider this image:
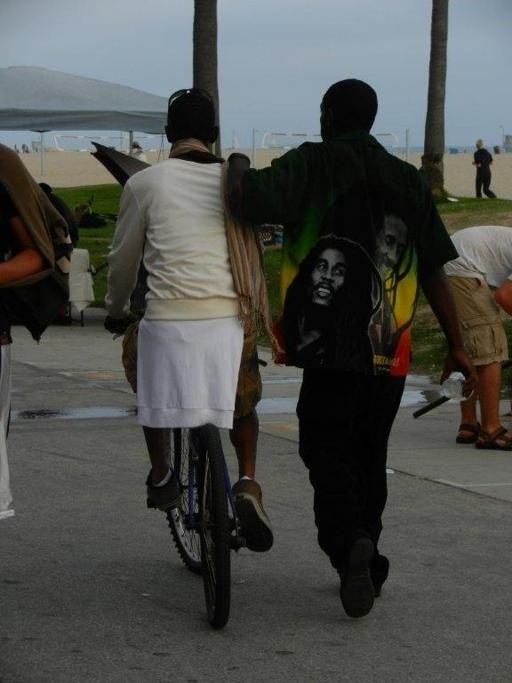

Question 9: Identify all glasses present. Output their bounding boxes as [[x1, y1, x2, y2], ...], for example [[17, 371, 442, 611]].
[[167, 87, 215, 111]]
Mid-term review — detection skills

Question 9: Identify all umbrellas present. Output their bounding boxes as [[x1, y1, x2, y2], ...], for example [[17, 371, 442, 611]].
[[0, 64, 183, 134]]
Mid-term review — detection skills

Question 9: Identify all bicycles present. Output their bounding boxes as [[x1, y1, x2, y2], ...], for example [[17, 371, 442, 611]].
[[104, 306, 248, 632]]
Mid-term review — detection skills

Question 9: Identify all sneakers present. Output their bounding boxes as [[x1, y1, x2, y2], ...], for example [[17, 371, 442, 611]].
[[230, 478, 273, 553], [337, 536, 390, 620], [145, 464, 182, 513]]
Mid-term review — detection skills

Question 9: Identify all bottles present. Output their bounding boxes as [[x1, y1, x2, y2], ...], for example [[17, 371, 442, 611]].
[[441, 377, 474, 401]]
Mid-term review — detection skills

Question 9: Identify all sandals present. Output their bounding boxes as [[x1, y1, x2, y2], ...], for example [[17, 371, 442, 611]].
[[456, 420, 512, 451]]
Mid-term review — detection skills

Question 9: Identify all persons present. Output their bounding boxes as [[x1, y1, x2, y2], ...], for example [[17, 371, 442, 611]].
[[94, 86, 277, 560], [469, 136, 496, 198], [272, 233, 386, 376], [37, 181, 81, 247], [336, 195, 414, 376], [221, 71, 479, 621], [130, 140, 148, 161], [432, 223, 512, 452], [1, 136, 70, 519]]
